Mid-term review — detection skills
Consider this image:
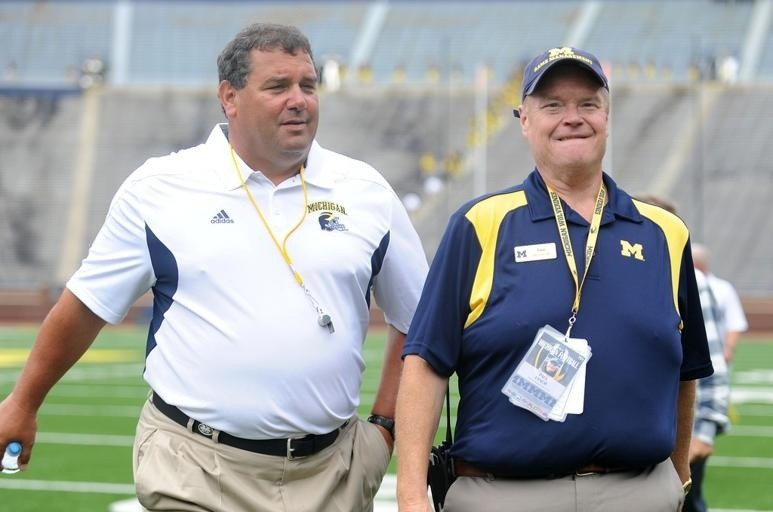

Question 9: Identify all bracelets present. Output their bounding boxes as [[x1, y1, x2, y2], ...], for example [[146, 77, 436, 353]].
[[364, 408, 396, 441], [678, 475, 693, 495]]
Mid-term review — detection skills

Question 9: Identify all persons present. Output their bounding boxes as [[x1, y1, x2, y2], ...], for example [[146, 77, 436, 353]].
[[629, 188, 734, 511], [392, 45, 715, 512], [540, 356, 562, 379], [0, 22, 430, 512], [692, 241, 749, 364]]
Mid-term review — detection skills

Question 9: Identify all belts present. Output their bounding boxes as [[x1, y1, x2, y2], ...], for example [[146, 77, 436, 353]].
[[153, 391, 351, 461], [456, 461, 631, 479]]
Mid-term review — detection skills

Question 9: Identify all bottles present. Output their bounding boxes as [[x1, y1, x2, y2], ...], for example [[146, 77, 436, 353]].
[[1, 440, 27, 474]]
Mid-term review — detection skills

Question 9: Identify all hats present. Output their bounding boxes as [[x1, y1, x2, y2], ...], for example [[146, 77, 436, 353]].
[[513, 47, 609, 118]]
